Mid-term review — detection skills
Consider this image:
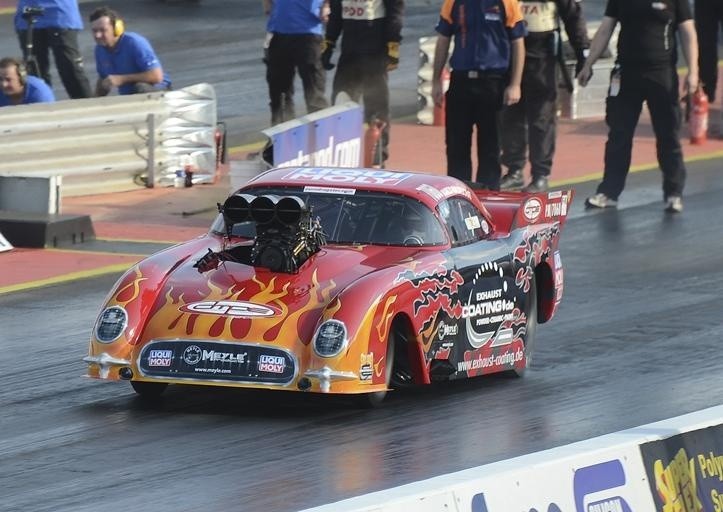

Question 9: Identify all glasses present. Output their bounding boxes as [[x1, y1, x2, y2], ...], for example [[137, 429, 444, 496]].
[[402, 219, 425, 232]]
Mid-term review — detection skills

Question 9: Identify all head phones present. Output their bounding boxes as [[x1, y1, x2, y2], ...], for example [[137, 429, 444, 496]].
[[113, 18, 126, 37]]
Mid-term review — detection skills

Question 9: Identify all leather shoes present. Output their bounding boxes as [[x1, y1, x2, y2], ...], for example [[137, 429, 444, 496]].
[[499, 175, 524, 191], [521, 176, 548, 193]]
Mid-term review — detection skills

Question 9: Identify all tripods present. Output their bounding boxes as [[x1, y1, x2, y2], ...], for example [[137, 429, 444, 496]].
[[17, 19, 42, 80]]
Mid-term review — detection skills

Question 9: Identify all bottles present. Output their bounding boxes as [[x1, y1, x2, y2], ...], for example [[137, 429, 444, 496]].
[[183, 153, 195, 189]]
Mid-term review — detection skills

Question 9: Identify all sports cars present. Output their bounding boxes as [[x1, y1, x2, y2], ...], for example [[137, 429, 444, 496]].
[[83, 165, 577, 409]]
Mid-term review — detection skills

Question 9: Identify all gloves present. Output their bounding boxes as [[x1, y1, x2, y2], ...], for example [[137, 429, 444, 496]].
[[385, 41, 400, 71], [319, 39, 334, 70], [575, 44, 593, 80]]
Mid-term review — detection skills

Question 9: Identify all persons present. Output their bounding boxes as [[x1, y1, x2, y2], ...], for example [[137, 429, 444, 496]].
[[430, 0, 529, 192], [14, 0, 94, 98], [499, 0, 594, 193], [0, 57, 55, 107], [322, 0, 406, 165], [262, 0, 331, 125], [87, 6, 171, 97], [577, 0, 700, 211]]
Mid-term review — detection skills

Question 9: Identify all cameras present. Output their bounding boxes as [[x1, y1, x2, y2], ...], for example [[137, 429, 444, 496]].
[[21, 4, 45, 20]]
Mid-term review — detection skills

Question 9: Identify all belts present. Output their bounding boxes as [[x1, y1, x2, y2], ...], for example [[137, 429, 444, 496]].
[[455, 70, 501, 80]]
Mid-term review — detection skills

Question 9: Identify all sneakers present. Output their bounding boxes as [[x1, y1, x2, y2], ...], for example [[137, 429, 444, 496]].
[[584, 193, 618, 208], [664, 195, 683, 212]]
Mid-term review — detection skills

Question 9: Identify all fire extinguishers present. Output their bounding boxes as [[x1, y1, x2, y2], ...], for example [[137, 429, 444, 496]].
[[212, 119, 229, 186], [688, 84, 709, 145], [362, 113, 387, 168], [432, 65, 451, 127]]
